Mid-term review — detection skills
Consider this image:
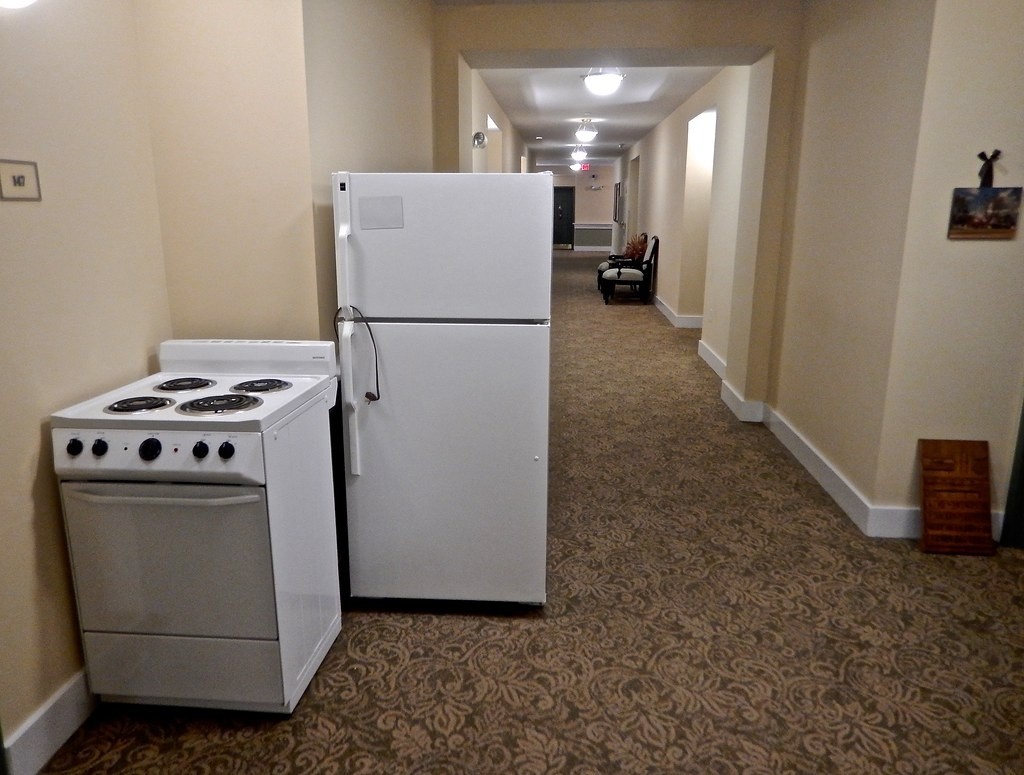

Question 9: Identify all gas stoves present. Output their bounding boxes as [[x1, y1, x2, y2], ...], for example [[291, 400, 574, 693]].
[[49, 339, 337, 486]]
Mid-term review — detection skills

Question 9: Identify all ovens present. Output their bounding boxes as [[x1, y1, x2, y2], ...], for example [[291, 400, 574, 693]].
[[59, 477, 342, 714]]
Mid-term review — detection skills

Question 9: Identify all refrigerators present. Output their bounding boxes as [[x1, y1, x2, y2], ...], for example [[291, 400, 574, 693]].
[[328, 170, 554, 606]]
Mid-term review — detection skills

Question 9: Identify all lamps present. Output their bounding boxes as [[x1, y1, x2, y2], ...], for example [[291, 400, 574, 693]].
[[571, 145, 588, 161], [575, 120, 599, 142], [475, 132, 488, 149], [582, 67, 627, 95]]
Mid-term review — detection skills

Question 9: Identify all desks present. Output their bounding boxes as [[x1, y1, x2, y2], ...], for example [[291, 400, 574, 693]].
[[607, 258, 635, 269]]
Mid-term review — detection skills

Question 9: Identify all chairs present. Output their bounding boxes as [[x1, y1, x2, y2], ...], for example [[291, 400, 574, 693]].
[[598, 232, 659, 306]]
